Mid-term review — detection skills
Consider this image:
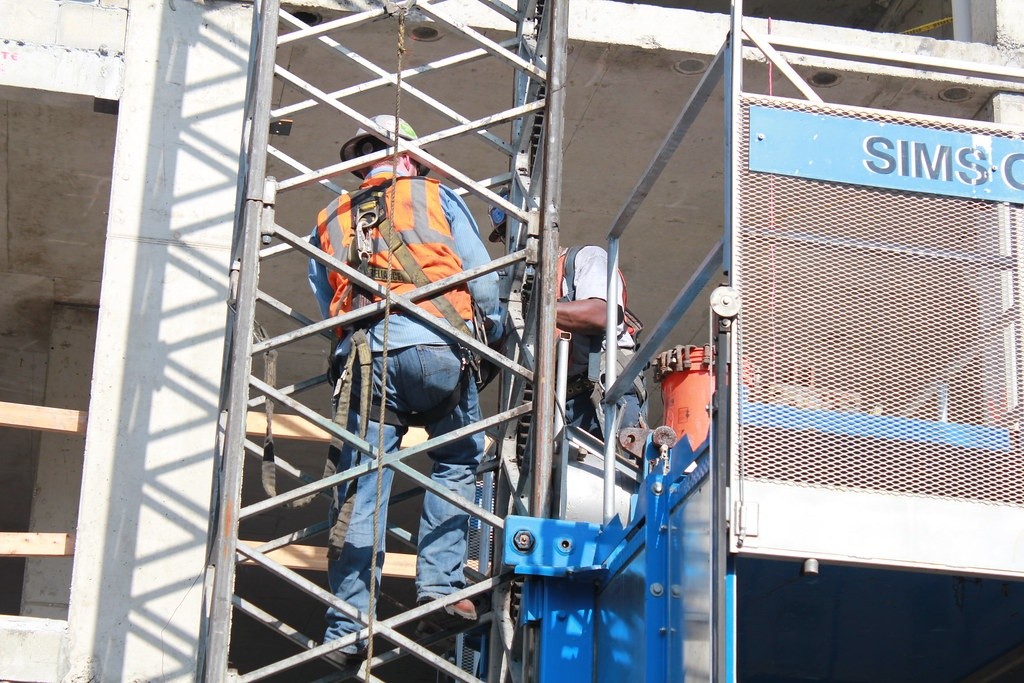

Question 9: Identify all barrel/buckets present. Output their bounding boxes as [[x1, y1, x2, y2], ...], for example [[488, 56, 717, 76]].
[[657, 347, 754, 450]]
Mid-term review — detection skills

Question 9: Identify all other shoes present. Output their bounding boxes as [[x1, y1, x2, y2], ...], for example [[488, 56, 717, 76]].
[[419, 594, 477, 631]]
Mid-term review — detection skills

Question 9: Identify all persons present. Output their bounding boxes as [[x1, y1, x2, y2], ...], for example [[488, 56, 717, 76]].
[[306, 114, 505, 664], [485, 180, 651, 458]]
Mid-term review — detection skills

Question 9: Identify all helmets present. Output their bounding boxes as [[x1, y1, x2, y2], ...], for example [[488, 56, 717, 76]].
[[340, 115, 430, 181], [487, 187, 558, 242]]
